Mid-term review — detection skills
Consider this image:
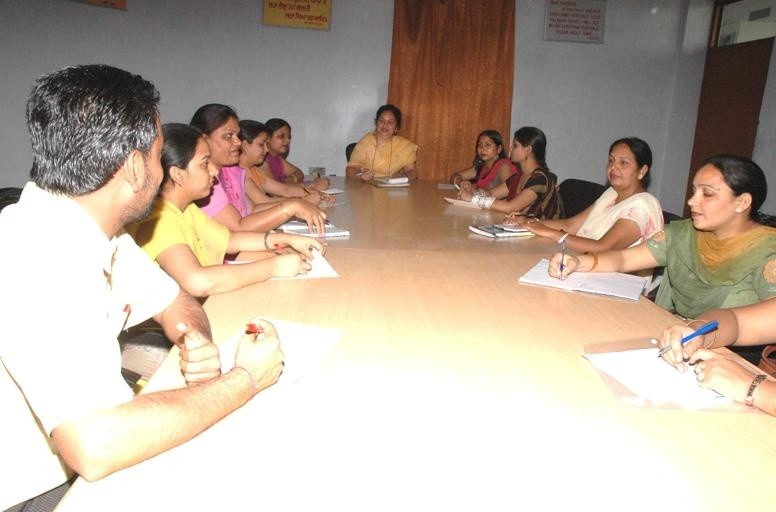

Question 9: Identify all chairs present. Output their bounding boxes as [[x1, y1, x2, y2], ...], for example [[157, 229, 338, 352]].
[[344, 142, 358, 161], [650, 210, 686, 301], [560, 178, 606, 233]]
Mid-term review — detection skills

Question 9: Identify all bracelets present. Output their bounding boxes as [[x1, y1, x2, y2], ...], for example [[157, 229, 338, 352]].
[[453, 175, 460, 188], [290, 173, 298, 183], [470, 193, 497, 210], [687, 319, 716, 349], [303, 179, 314, 191], [558, 230, 570, 245], [534, 217, 540, 223], [744, 373, 768, 407], [264, 231, 272, 251], [582, 250, 600, 273], [346, 104, 418, 183], [231, 366, 257, 400]]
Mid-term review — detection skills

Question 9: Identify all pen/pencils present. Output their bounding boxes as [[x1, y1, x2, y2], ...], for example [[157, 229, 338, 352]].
[[560, 240, 565, 280], [514, 214, 534, 217], [299, 219, 329, 224], [303, 187, 310, 194], [274, 244, 290, 254], [247, 324, 264, 332], [656, 320, 720, 357]]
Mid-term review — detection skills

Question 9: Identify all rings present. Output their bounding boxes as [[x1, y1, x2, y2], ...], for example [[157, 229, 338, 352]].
[[527, 221, 532, 224]]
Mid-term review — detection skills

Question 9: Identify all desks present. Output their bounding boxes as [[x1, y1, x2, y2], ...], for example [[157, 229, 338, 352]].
[[57, 169, 776, 511]]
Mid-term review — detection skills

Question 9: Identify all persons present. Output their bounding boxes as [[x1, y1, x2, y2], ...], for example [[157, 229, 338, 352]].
[[501, 136, 665, 295], [549, 154, 776, 364], [2, 66, 284, 512], [263, 114, 330, 191], [191, 103, 328, 237], [457, 127, 564, 219], [451, 130, 522, 201], [657, 297, 776, 416], [124, 122, 327, 380], [238, 118, 335, 211]]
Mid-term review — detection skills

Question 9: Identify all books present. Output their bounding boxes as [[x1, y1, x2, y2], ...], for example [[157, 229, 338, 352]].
[[469, 223, 540, 238], [278, 219, 350, 239], [370, 175, 413, 189]]
